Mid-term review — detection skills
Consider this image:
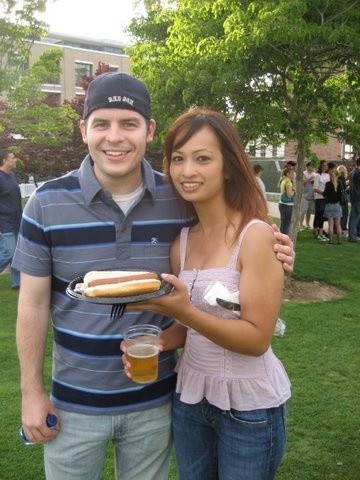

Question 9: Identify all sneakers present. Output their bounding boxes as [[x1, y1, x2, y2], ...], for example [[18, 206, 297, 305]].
[[315, 236, 326, 241], [321, 235, 330, 241]]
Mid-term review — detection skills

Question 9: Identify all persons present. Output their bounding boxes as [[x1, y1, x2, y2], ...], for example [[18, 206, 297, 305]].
[[323, 170, 345, 243], [0, 150, 24, 291], [126, 109, 288, 480], [339, 166, 349, 230], [300, 159, 316, 231], [313, 160, 328, 240], [253, 164, 267, 203], [328, 161, 334, 175], [14, 72, 295, 480], [278, 166, 295, 236], [349, 161, 360, 243], [283, 156, 299, 182]]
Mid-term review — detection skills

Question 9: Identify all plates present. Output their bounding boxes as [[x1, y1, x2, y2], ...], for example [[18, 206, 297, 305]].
[[65, 267, 173, 306]]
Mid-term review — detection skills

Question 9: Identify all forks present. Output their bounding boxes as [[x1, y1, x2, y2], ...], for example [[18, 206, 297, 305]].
[[110, 305, 125, 321]]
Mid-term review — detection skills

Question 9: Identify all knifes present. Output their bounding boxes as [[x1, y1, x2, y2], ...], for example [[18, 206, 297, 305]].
[[216, 297, 241, 311]]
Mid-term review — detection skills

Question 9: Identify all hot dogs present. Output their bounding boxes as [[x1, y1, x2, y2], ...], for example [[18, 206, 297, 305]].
[[83, 270, 162, 299]]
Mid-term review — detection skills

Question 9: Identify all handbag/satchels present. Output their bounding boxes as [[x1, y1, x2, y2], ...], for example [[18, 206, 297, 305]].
[[281, 181, 295, 202], [339, 180, 350, 204]]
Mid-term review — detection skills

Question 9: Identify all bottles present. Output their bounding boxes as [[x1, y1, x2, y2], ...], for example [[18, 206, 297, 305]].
[[19, 415, 57, 446]]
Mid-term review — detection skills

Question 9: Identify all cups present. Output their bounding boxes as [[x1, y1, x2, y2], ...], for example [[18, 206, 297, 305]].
[[120, 324, 162, 385]]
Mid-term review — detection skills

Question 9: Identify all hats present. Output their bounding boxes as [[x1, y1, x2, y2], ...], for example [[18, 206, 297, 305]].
[[83, 72, 152, 120]]
